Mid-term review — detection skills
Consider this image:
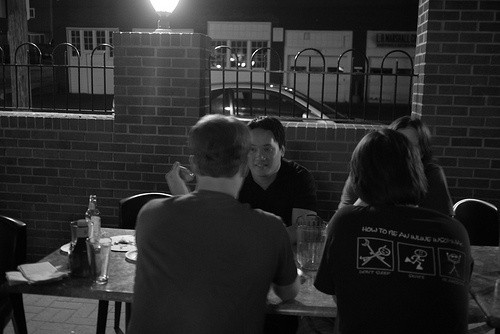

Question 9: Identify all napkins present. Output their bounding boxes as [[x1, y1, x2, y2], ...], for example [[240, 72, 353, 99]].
[[5, 262, 71, 284]]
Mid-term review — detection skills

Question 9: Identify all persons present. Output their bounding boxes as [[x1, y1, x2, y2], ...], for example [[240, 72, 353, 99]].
[[314, 128, 475, 333], [326, 116, 454, 239], [126, 115, 300, 333], [238, 116, 317, 243]]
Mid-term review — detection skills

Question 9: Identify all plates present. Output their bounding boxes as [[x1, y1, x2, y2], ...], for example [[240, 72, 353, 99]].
[[60, 242, 70, 252], [125, 250, 138, 262], [100, 234, 138, 251]]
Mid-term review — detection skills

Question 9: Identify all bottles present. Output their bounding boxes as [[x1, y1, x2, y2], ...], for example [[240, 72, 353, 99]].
[[67, 219, 95, 281], [85, 195, 101, 245]]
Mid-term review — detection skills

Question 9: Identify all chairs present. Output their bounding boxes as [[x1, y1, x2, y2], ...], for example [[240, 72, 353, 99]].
[[111, 193, 173, 329], [453, 198, 500, 247]]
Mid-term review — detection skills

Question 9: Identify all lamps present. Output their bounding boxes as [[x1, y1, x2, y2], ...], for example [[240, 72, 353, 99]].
[[150, 0, 179, 28]]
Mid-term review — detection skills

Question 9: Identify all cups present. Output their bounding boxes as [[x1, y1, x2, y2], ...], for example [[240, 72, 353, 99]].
[[297, 225, 328, 270], [90, 236, 113, 284], [69, 221, 94, 254]]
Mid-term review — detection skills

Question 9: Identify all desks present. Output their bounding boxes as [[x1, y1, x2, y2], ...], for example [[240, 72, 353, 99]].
[[14, 228, 500, 334]]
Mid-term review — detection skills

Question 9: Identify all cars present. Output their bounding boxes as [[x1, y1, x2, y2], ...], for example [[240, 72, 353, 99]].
[[209, 82, 353, 123]]
